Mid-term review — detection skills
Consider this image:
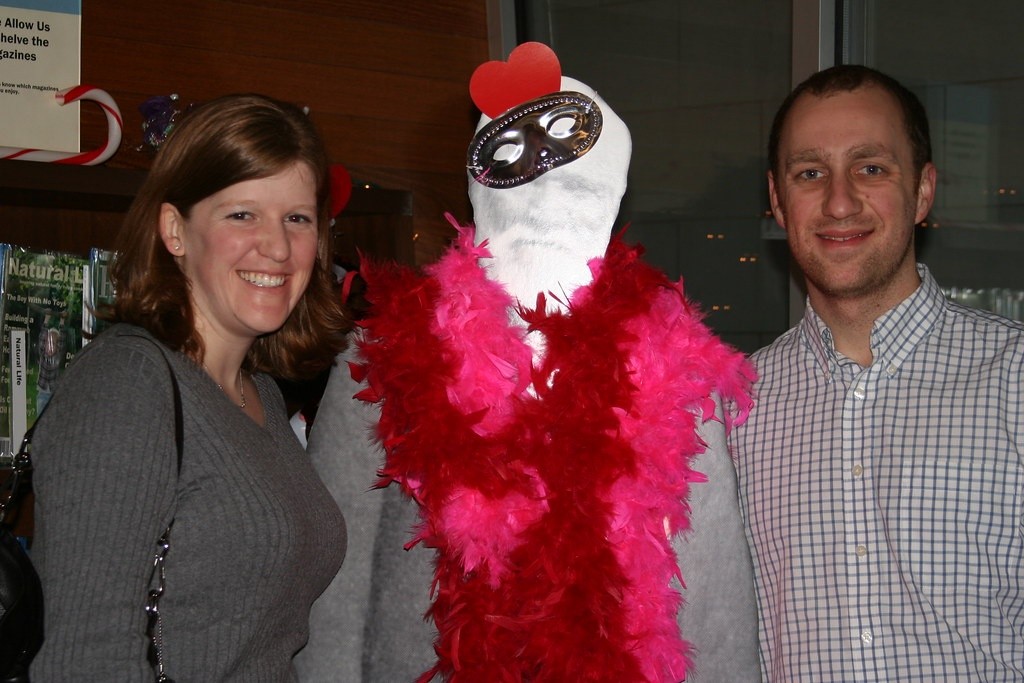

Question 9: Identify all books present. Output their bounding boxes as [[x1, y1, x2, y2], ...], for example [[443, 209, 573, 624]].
[[0, 243, 121, 468]]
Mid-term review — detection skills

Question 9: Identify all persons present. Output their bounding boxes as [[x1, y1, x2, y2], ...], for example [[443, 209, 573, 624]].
[[14, 93, 348, 683], [720, 64, 1024, 683], [288, 42, 761, 682], [36, 308, 69, 418]]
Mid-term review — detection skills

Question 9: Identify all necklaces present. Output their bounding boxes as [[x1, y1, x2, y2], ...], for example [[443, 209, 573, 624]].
[[202, 361, 246, 409]]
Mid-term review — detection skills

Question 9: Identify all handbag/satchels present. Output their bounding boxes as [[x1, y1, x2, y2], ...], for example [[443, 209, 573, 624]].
[[0, 334, 184, 683]]
